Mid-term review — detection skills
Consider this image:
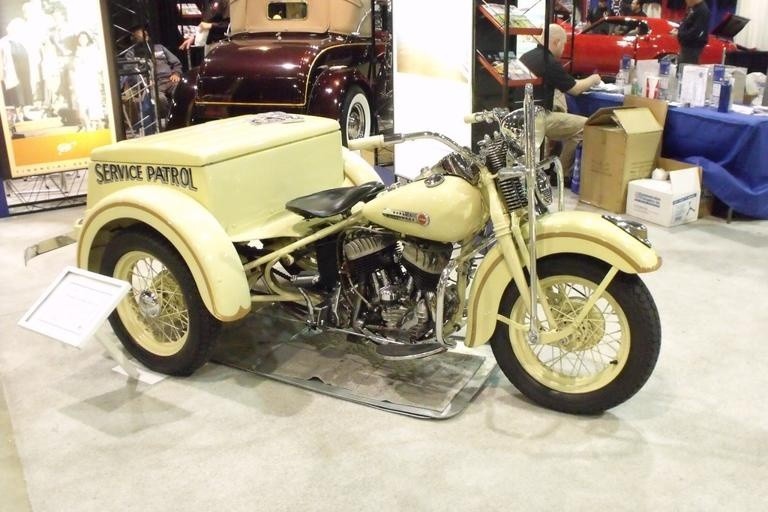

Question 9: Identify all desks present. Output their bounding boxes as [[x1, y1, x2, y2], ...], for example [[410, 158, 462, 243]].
[[562, 83, 766, 225]]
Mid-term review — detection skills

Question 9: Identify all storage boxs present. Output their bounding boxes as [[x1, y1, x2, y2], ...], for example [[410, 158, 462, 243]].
[[623, 157, 703, 226], [578, 93, 668, 216], [695, 185, 713, 218]]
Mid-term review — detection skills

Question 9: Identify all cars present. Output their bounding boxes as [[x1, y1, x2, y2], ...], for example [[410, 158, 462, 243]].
[[550, 13, 756, 79], [166, 1, 392, 147]]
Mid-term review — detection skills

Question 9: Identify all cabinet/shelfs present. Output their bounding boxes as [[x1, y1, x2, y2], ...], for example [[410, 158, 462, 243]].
[[474, 0, 544, 160]]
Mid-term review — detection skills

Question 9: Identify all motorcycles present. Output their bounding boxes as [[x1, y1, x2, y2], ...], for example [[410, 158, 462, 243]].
[[73, 79, 668, 420]]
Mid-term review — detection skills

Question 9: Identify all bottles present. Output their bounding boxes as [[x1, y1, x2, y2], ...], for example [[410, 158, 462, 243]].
[[630, 79, 639, 95], [718, 79, 732, 113], [616, 68, 625, 87]]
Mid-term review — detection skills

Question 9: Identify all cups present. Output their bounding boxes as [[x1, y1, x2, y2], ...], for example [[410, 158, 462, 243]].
[[644, 74, 661, 100]]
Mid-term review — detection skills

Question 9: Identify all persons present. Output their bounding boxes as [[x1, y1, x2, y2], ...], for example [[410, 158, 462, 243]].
[[122, 24, 185, 121], [178, 1, 229, 55], [501, 23, 602, 188], [618, 0, 650, 36], [591, 0, 616, 35], [553, 0, 571, 24], [0, 0, 111, 136], [675, 0, 710, 78]]
